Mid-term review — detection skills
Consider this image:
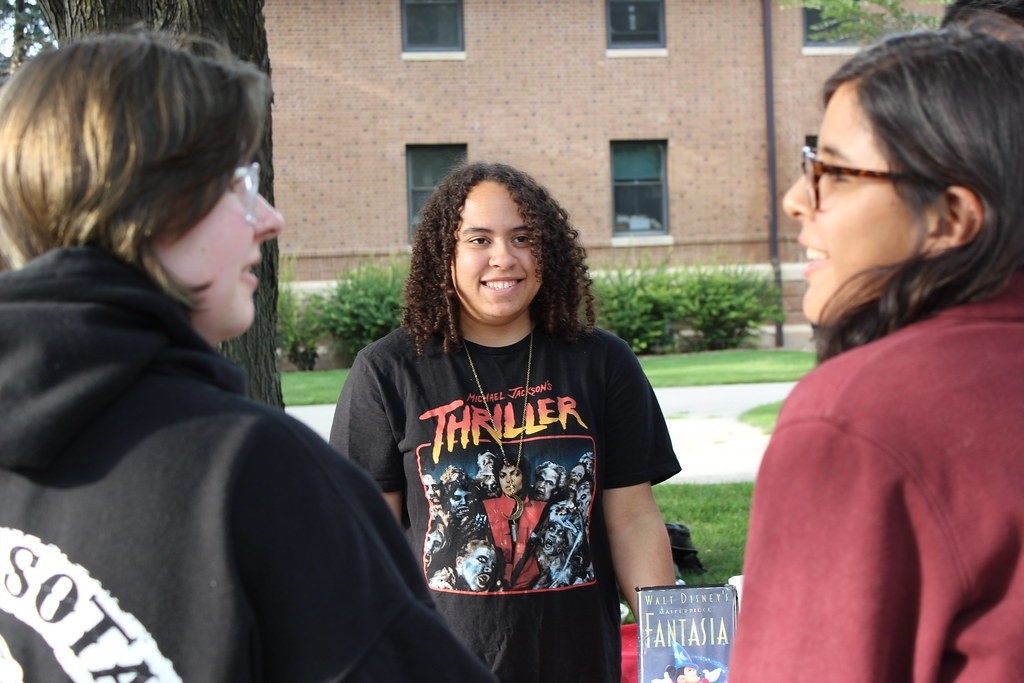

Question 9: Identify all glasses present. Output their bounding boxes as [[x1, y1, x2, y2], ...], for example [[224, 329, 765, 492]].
[[218, 162, 259, 223], [800, 145, 916, 211]]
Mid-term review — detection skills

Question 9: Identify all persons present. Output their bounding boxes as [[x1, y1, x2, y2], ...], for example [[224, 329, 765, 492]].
[[328, 162, 681, 683], [0, 35, 502, 683], [732, 0, 1024, 683]]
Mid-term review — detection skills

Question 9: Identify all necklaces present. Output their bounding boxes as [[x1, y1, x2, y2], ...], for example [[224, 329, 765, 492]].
[[462, 331, 533, 542]]
